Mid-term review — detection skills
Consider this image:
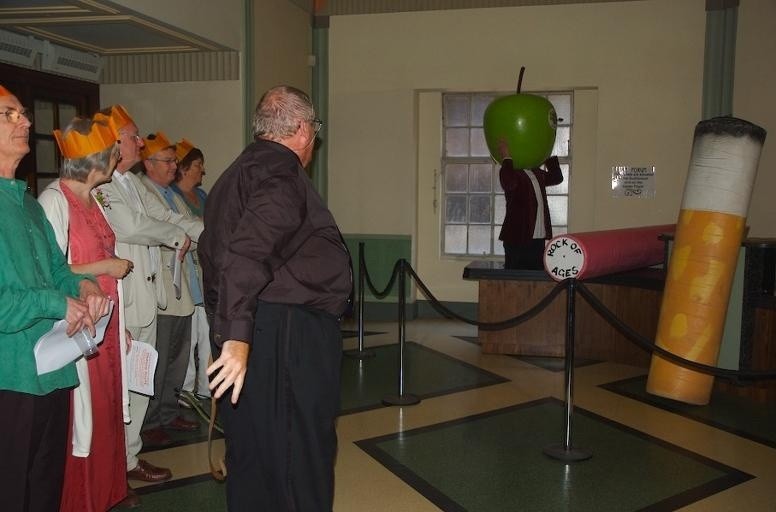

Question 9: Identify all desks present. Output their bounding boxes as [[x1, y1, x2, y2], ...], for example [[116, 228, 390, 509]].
[[463, 233, 776, 383]]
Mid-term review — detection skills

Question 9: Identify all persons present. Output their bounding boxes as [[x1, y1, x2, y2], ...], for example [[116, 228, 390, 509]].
[[90, 104, 204, 508], [197, 84, 353, 512], [498, 140, 563, 271], [0, 85, 110, 512], [169, 137, 212, 409], [135, 133, 204, 444], [38, 111, 134, 512]]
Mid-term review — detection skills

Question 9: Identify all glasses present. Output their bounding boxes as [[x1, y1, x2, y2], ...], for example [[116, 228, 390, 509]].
[[151, 157, 178, 165], [309, 117, 323, 133], [0, 107, 34, 124], [128, 133, 143, 142]]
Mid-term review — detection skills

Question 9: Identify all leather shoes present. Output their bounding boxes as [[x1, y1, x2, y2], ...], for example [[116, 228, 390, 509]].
[[121, 483, 142, 508], [168, 418, 200, 431], [128, 459, 172, 482], [143, 429, 169, 445]]
